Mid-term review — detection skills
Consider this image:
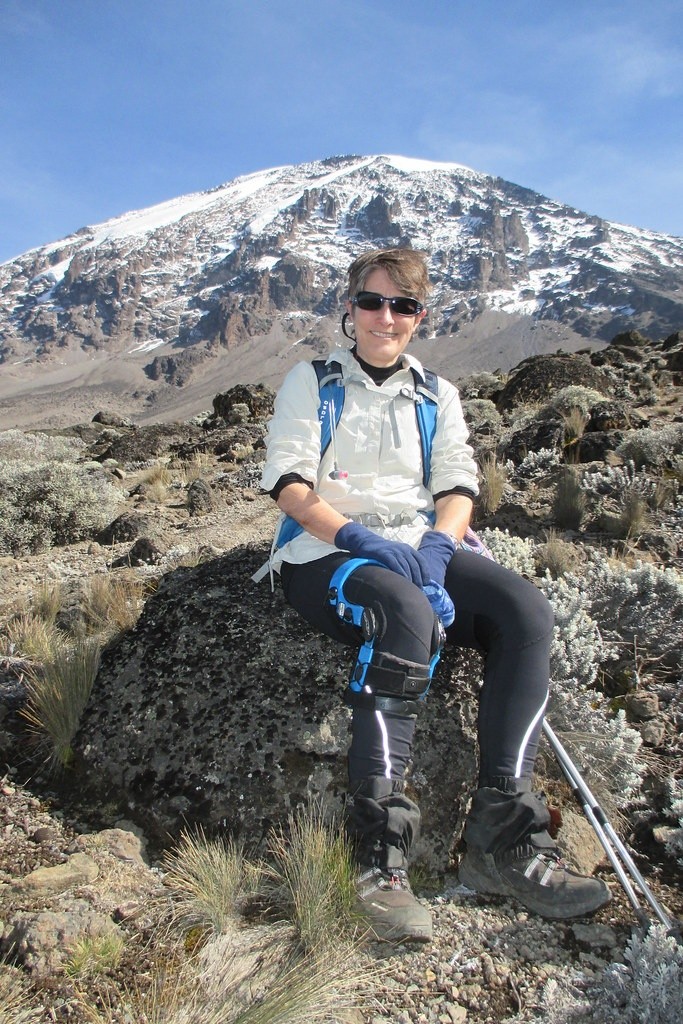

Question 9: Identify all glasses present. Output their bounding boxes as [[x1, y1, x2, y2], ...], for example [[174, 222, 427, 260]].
[[349, 291, 424, 317]]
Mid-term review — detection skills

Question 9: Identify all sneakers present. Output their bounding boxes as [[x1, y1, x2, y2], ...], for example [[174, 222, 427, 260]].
[[349, 864, 433, 943], [457, 846, 613, 920]]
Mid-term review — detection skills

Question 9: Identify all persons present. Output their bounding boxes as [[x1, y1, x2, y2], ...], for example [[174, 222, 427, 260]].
[[260, 246, 611, 941]]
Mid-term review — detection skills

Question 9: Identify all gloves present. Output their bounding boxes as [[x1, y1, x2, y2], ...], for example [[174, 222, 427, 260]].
[[334, 522, 429, 592], [417, 530, 457, 590]]
